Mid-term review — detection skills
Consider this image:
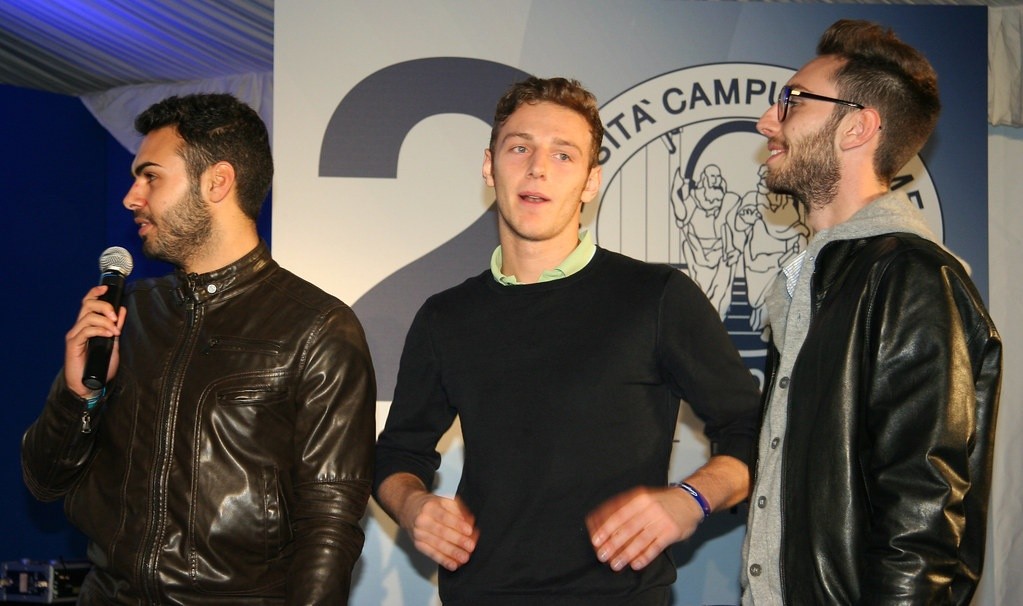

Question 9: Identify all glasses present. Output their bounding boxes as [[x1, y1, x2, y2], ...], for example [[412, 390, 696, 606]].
[[777, 84, 882, 130]]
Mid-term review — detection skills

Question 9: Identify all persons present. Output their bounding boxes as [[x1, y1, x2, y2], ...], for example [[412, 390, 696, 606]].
[[738, 17, 1003, 606], [22, 91, 378, 605], [371, 75, 763, 606]]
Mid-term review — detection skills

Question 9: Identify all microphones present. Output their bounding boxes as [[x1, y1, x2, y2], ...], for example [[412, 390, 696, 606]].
[[80, 246, 134, 391]]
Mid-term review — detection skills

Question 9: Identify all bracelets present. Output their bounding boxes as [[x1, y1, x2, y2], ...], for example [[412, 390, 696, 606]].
[[676, 481, 711, 524]]
